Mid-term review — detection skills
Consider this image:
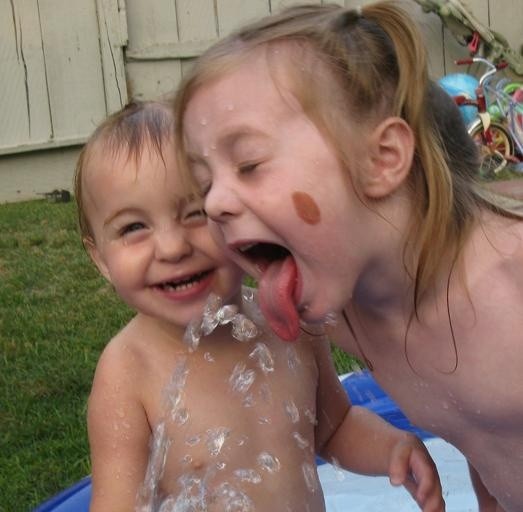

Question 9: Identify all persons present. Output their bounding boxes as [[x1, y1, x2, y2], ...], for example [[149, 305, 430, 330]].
[[73, 100, 446, 512], [173, 0, 523, 512]]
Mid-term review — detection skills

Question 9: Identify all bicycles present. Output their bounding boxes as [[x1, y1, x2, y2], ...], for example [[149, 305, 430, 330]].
[[448, 50, 523, 177]]
